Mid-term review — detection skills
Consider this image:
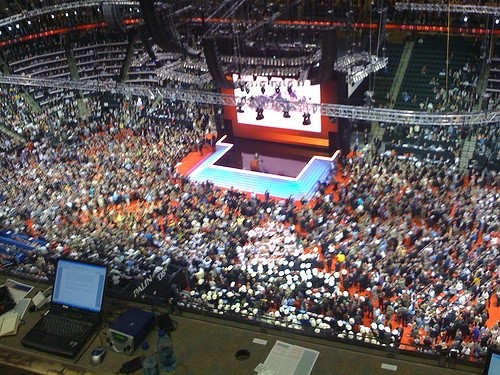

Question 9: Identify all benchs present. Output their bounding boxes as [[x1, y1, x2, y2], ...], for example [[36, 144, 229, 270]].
[[0, 1, 499, 170]]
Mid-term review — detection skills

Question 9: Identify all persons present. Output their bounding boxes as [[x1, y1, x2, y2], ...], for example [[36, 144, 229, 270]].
[[3, 45, 500, 358], [232, 73, 314, 125]]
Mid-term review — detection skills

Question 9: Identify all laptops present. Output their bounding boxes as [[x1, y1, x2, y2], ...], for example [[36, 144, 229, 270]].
[[21, 255, 109, 360]]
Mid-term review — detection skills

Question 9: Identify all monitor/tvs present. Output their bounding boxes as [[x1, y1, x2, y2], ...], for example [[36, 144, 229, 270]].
[[483, 349, 500, 375]]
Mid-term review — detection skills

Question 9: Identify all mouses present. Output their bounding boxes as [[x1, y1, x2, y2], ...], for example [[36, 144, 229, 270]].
[[90, 345, 107, 366]]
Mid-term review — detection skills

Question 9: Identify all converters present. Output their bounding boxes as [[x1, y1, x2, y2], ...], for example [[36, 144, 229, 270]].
[[119, 354, 149, 375]]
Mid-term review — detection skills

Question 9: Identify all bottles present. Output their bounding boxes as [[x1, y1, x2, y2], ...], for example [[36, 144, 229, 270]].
[[140, 342, 160, 375], [157, 329, 177, 372]]
